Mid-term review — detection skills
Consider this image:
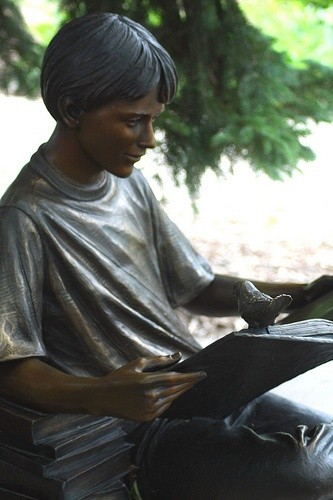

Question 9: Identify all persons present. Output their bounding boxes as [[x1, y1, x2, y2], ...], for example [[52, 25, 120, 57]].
[[0, 11, 333, 499]]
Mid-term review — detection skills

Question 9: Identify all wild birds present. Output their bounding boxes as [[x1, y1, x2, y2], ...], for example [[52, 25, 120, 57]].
[[232, 279, 293, 335]]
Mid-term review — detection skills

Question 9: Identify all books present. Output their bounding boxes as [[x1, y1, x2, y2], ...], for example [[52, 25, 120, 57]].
[[149, 290, 333, 420]]
